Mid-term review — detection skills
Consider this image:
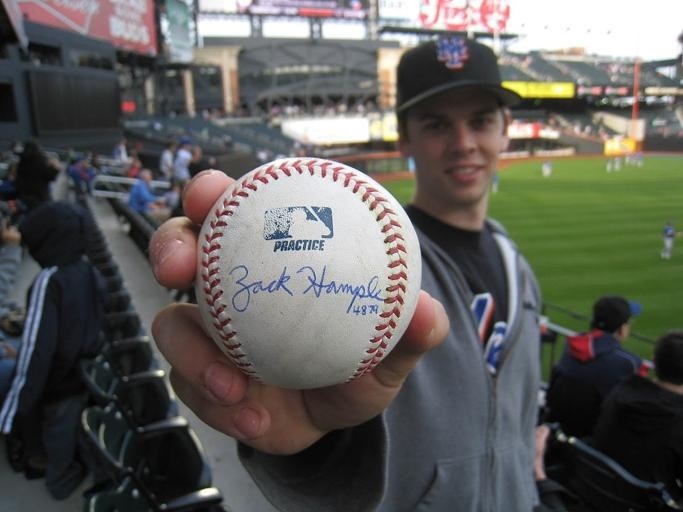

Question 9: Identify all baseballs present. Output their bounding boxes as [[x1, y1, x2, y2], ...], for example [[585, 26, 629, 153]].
[[193, 157, 423, 391]]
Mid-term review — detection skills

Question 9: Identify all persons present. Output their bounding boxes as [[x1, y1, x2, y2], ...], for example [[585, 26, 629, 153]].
[[1, 98, 381, 511], [543, 296, 651, 427], [661, 220, 682, 260], [149, 36, 543, 512], [493, 149, 646, 193], [591, 331, 683, 511]]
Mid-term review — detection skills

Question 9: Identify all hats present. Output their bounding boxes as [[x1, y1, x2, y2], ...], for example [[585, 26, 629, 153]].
[[395, 34, 523, 116], [592, 296, 642, 332]]
